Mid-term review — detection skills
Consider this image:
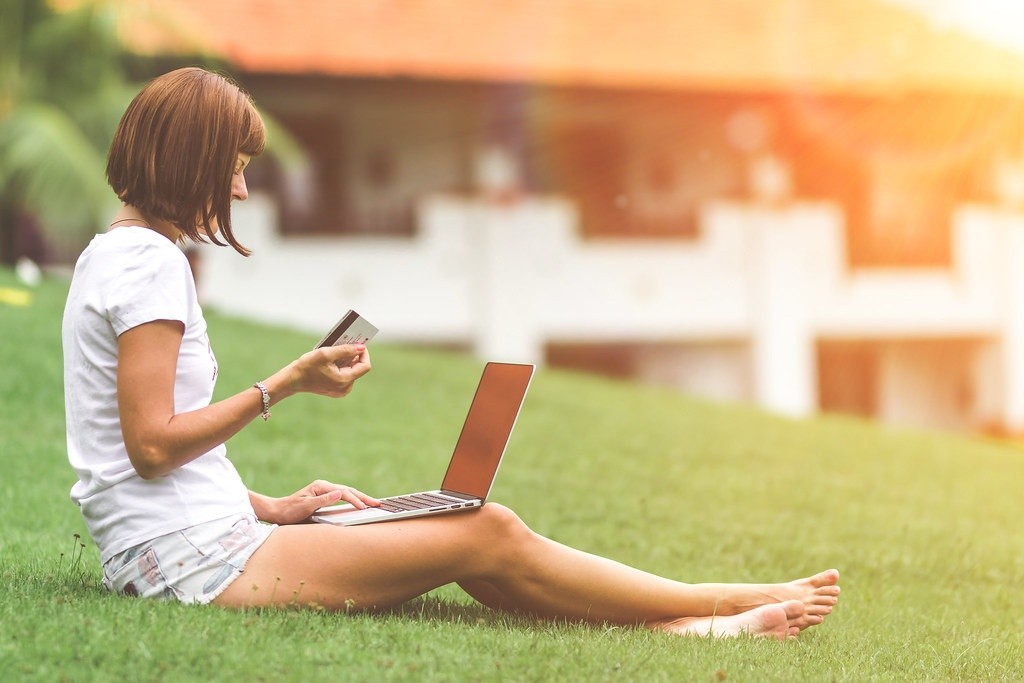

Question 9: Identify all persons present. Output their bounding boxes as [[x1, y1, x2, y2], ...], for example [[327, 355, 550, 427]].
[[61, 65, 840, 637]]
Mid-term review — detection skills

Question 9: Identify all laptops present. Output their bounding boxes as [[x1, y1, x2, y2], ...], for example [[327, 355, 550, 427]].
[[299, 361, 537, 525]]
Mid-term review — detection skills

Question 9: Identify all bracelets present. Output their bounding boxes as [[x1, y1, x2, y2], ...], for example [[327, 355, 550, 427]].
[[255, 382, 270, 421]]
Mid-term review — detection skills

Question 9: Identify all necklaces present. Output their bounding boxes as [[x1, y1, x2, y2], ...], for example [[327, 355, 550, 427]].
[[111, 218, 150, 228]]
